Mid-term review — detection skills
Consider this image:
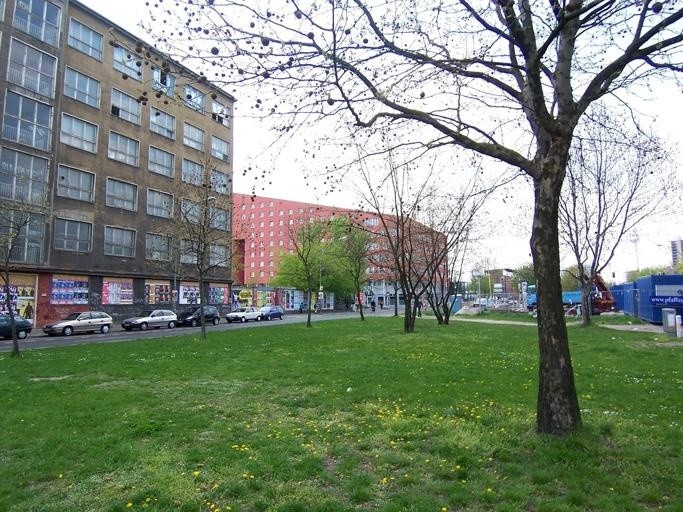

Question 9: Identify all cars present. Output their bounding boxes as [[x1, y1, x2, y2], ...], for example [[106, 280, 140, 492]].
[[471, 298, 487, 307], [43, 311, 113, 336], [225, 305, 284, 322], [0, 315, 32, 339], [122, 306, 220, 330]]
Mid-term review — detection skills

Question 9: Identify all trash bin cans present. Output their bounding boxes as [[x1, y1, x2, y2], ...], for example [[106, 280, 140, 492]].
[[662, 308, 676, 332]]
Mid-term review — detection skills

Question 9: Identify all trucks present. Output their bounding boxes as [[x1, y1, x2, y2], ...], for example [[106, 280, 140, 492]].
[[527, 285, 582, 314]]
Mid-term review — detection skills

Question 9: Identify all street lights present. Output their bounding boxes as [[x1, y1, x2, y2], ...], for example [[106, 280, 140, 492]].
[[319, 235, 347, 292]]
